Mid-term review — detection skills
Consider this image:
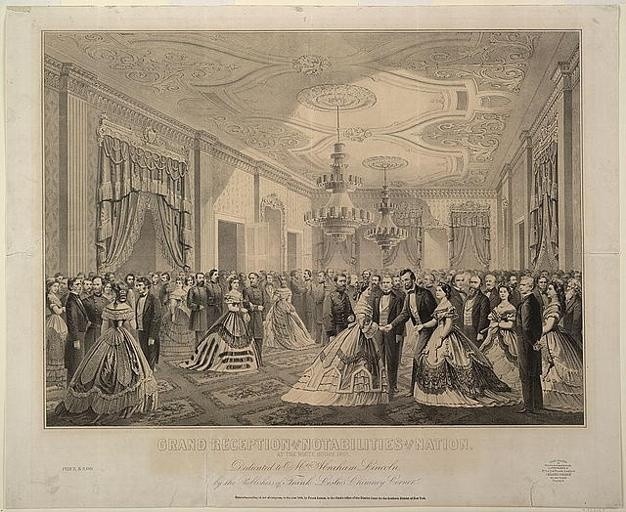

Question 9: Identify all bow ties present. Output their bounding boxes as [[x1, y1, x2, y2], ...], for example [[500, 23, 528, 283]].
[[407, 290, 414, 294], [381, 291, 391, 295], [139, 295, 145, 297]]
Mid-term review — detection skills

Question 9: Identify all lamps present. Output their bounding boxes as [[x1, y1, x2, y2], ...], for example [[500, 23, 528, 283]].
[[303, 106, 375, 244], [364, 168, 412, 254]]
